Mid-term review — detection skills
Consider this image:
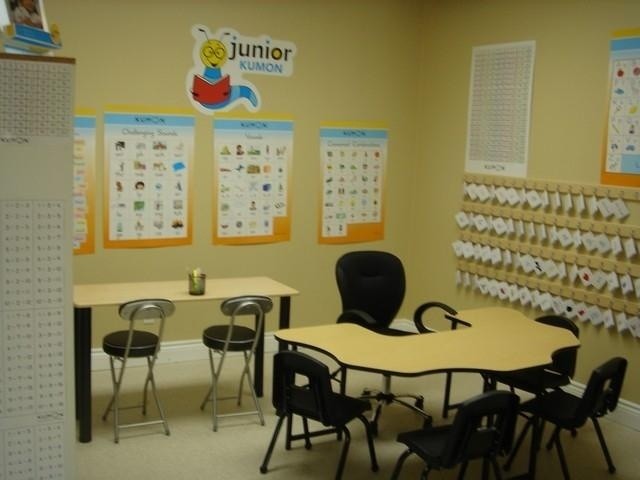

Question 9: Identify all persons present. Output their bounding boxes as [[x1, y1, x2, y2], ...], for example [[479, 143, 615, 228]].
[[9, 0, 43, 26]]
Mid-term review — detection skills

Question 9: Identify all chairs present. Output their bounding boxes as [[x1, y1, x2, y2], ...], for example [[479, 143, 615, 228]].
[[262, 347, 381, 480], [200, 295, 273, 432], [491, 311, 583, 444], [329, 249, 439, 431], [102, 299, 176, 444], [391, 389, 523, 479], [516, 354, 627, 479]]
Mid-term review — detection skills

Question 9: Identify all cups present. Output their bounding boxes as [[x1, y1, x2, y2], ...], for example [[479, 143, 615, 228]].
[[189, 273, 207, 295]]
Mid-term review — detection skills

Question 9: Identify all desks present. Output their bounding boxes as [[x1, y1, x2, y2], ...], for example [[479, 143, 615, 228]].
[[72, 277, 300, 443], [272, 302, 581, 458]]
